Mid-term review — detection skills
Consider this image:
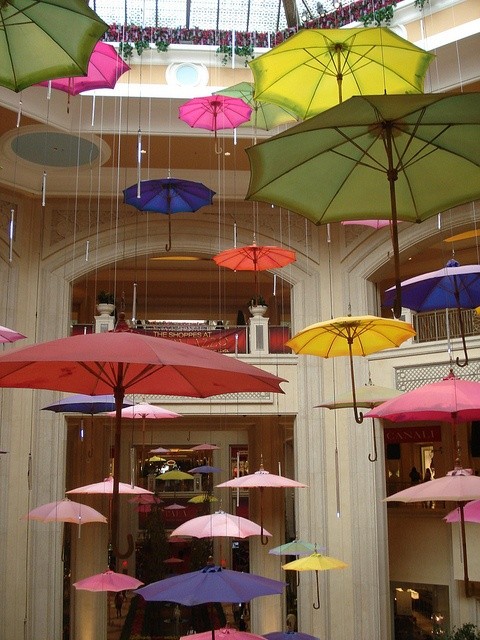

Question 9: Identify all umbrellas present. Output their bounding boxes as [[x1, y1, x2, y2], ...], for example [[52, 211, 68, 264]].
[[156, 470, 190, 495], [441, 500, 480, 523], [47, 42, 133, 112], [73, 571, 141, 592], [177, 625, 263, 639], [165, 503, 185, 510], [212, 81, 300, 148], [0, 331, 292, 557], [1, 325, 29, 344], [136, 505, 162, 513], [145, 446, 171, 466], [166, 558, 186, 565], [214, 471, 307, 544], [382, 471, 480, 597], [315, 382, 403, 463], [381, 261, 479, 367], [64, 476, 154, 501], [267, 630, 321, 640], [345, 219, 398, 254], [282, 315, 418, 422], [0, 322, 27, 343], [364, 374, 479, 423], [189, 465, 226, 494], [190, 444, 223, 450], [280, 554, 348, 612], [167, 510, 272, 542], [168, 537, 190, 546], [179, 95, 247, 155], [22, 497, 108, 530], [121, 177, 217, 253], [139, 565, 286, 639], [186, 495, 219, 504], [1, 1, 111, 91], [246, 92, 478, 316], [270, 538, 322, 589], [129, 494, 163, 514], [215, 240, 298, 310], [145, 455, 165, 464], [251, 25, 436, 122]]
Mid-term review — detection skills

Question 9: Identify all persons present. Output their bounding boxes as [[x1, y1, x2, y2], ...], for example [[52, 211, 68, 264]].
[[121, 590, 129, 598], [111, 592, 122, 620], [423, 468, 437, 508], [410, 467, 417, 484]]
